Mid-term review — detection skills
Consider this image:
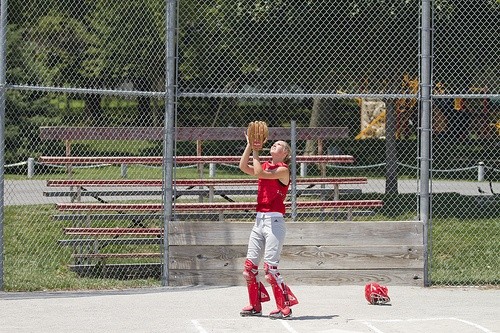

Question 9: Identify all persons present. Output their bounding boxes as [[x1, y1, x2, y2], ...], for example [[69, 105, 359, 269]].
[[239, 120, 299, 319]]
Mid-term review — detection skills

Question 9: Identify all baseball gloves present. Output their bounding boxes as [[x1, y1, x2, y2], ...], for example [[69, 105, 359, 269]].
[[244, 121, 269, 152]]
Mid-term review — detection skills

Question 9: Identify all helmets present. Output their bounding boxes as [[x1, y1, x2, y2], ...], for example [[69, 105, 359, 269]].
[[366, 283, 390, 304]]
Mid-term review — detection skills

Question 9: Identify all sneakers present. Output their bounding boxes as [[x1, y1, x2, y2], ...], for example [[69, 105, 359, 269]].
[[269, 309, 293, 320], [241, 305, 262, 316]]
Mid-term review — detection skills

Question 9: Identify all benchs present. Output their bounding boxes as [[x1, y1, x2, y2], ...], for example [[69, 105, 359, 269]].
[[39, 124, 385, 277]]
